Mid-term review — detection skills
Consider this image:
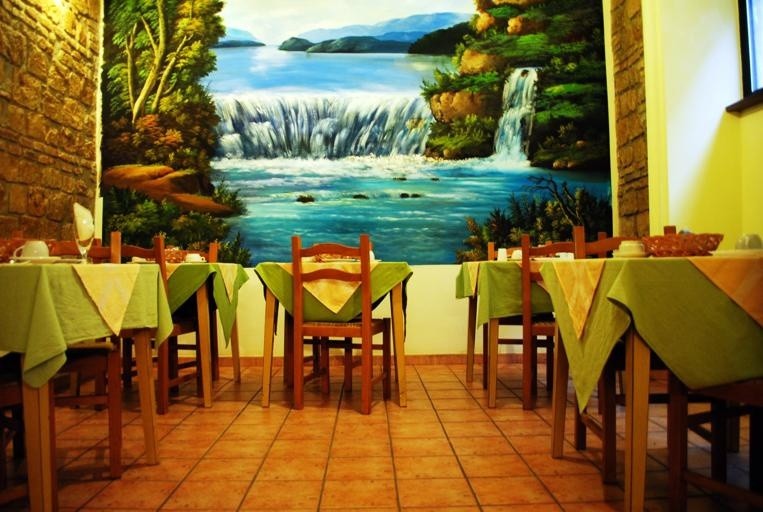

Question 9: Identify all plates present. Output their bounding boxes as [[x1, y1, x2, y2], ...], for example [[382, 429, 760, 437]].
[[8, 256, 63, 264], [134, 260, 155, 264], [356, 259, 381, 262], [320, 258, 355, 263], [184, 260, 207, 263], [612, 252, 649, 258], [708, 249, 762, 259], [61, 258, 82, 263]]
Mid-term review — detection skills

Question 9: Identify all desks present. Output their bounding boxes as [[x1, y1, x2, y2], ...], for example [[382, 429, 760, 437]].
[[255, 257, 412, 410]]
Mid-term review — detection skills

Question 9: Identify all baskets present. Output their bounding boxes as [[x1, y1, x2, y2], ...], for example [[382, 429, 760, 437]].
[[641, 232, 724, 256], [146, 249, 201, 263]]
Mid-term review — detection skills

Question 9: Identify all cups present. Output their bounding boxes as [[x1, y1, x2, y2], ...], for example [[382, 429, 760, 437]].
[[497, 248, 507, 262], [12, 240, 49, 257], [617, 240, 645, 254], [369, 250, 375, 260], [186, 253, 205, 261], [735, 233, 762, 250], [511, 249, 522, 259]]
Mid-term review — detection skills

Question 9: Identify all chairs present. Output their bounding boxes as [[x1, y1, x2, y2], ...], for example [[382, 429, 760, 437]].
[[311, 240, 374, 393], [289, 234, 393, 415]]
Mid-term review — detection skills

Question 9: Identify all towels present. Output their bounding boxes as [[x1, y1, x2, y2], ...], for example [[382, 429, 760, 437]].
[[72, 202, 96, 243]]
